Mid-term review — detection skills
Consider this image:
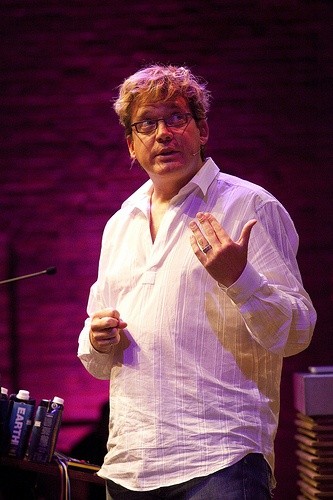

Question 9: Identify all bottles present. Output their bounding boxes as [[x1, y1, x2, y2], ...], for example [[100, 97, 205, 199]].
[[0, 386, 64, 463]]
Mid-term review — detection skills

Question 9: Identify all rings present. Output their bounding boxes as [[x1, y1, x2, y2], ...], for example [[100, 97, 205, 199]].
[[203, 245, 212, 253]]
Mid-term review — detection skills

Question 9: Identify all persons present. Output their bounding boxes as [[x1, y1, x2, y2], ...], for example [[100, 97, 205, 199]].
[[77, 65, 317, 500]]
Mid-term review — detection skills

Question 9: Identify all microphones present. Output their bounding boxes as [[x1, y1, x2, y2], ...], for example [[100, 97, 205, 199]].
[[193, 149, 201, 157], [0, 267, 58, 285]]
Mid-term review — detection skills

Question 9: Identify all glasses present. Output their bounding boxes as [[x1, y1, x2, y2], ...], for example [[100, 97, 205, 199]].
[[127, 112, 193, 133]]
